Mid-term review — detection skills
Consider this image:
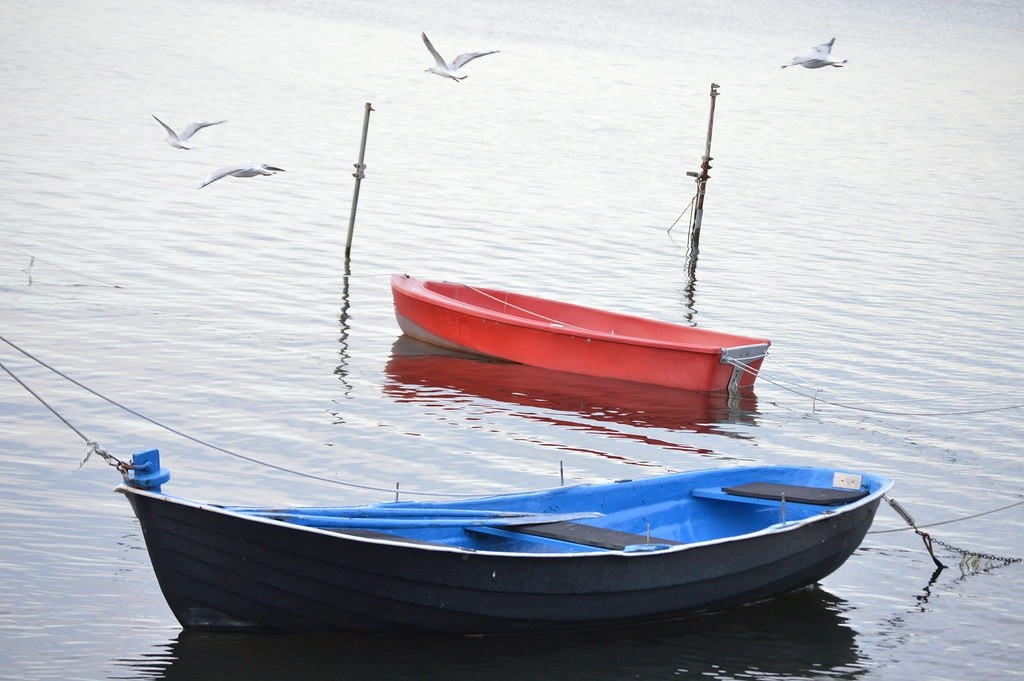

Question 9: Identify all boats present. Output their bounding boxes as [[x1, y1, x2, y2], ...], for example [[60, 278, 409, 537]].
[[113, 450, 894, 631], [391, 272, 776, 393]]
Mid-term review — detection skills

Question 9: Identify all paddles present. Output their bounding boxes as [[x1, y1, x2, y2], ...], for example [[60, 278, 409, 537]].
[[230, 510, 606, 529]]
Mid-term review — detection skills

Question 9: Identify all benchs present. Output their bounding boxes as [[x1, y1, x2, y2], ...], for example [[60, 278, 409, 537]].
[[466, 514, 684, 553], [693, 480, 869, 514]]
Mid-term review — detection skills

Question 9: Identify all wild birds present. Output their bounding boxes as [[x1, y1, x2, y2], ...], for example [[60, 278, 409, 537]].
[[780, 36, 847, 71], [420, 30, 500, 82], [198, 162, 285, 189], [151, 113, 228, 150]]
